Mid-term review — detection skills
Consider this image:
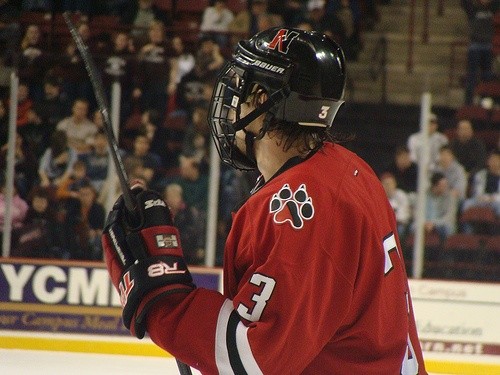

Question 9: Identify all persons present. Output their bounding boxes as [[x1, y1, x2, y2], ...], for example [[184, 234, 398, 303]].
[[0, 0, 379, 267], [379, 0, 500, 271], [102, 26, 428, 375]]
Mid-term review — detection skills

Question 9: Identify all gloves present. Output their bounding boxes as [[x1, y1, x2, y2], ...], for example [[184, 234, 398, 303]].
[[103, 186, 196, 339]]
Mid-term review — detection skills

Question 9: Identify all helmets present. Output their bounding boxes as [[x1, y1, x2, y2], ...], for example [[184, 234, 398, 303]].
[[231, 26, 347, 129]]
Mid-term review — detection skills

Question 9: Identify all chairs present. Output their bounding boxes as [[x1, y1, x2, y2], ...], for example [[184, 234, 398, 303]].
[[0, 0, 500, 283]]
